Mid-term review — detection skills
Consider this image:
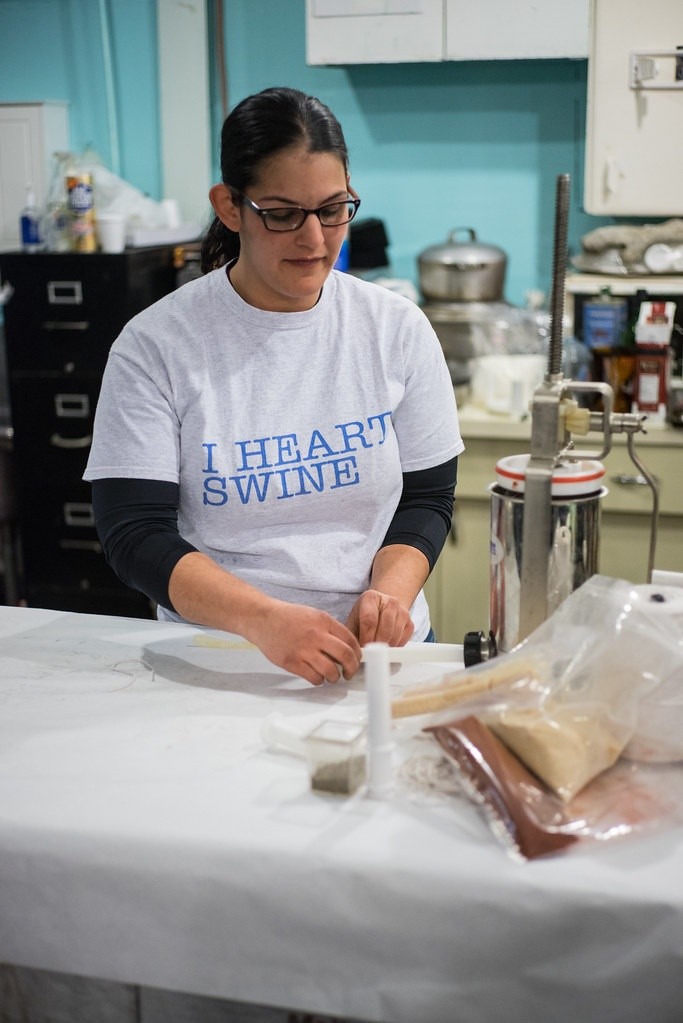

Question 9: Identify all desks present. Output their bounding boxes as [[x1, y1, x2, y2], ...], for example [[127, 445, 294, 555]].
[[0, 604, 683, 1023]]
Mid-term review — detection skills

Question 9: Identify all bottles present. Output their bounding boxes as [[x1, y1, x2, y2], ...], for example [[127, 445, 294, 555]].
[[64, 171, 97, 251], [19, 184, 42, 251]]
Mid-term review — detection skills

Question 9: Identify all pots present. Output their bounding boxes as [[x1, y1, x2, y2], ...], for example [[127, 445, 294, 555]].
[[414, 226, 521, 386]]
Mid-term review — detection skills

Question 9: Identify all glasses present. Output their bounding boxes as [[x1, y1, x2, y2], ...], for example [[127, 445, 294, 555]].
[[229, 183, 361, 232]]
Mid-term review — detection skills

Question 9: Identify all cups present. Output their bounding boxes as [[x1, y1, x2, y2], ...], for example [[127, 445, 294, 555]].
[[93, 212, 127, 254]]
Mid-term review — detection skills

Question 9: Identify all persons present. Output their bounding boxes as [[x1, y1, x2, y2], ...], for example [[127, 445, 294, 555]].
[[77, 85, 466, 686]]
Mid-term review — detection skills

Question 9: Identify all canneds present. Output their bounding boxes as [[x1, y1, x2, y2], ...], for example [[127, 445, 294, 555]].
[[64, 171, 96, 254]]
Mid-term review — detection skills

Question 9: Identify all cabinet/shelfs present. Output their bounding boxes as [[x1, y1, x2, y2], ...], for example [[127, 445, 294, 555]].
[[0, 241, 200, 619], [307, 0, 590, 67]]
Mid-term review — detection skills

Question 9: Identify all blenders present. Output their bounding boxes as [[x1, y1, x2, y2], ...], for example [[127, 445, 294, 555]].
[[465, 457, 604, 669]]
